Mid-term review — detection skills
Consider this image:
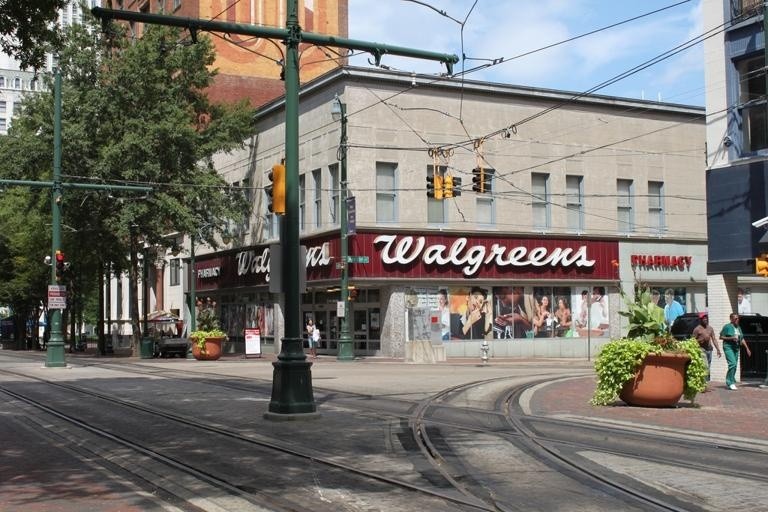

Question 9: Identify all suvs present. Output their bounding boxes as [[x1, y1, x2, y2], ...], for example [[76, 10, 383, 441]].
[[669, 313, 768, 375]]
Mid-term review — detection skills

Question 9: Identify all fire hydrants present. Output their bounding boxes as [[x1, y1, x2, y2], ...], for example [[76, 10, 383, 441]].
[[481, 338, 489, 364]]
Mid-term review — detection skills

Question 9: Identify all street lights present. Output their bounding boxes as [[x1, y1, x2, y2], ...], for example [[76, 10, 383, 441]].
[[44, 50, 68, 367], [328, 93, 355, 361]]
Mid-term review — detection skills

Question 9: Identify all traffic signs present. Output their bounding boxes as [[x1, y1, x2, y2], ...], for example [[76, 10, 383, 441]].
[[347, 256, 369, 264]]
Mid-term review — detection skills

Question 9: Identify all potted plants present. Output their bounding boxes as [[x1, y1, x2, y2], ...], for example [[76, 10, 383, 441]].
[[588, 275, 709, 408], [189, 306, 230, 360]]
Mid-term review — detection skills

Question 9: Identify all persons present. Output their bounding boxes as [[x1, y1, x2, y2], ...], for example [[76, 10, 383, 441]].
[[246, 307, 255, 329], [435, 286, 609, 340], [651, 290, 661, 305], [692, 314, 722, 384], [737, 286, 751, 315], [664, 289, 684, 332], [256, 307, 264, 334], [719, 313, 752, 390], [307, 319, 318, 359]]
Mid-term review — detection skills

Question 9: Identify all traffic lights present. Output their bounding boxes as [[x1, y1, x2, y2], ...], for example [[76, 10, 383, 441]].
[[483, 168, 495, 193], [425, 175, 434, 197], [265, 164, 285, 214], [471, 168, 480, 193], [56, 252, 65, 278], [756, 254, 768, 276], [452, 176, 463, 200]]
